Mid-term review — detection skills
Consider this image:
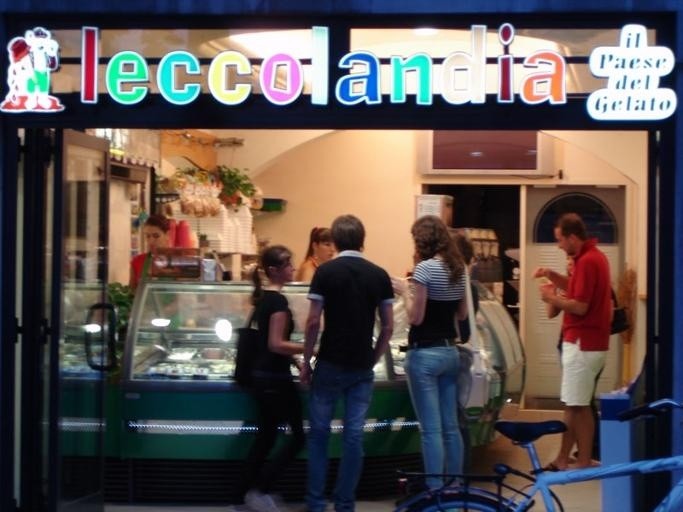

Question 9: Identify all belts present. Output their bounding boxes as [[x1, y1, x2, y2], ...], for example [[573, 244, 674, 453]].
[[399, 339, 456, 352]]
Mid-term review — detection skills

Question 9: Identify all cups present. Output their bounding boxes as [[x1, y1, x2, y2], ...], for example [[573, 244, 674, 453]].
[[541, 283, 555, 292], [202, 257, 216, 281], [167, 217, 192, 247]]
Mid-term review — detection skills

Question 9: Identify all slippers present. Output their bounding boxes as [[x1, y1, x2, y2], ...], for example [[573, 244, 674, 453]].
[[530, 463, 559, 476]]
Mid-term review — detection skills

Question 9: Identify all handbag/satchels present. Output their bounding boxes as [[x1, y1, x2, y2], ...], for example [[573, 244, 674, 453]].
[[608, 307, 629, 334], [231, 327, 259, 378]]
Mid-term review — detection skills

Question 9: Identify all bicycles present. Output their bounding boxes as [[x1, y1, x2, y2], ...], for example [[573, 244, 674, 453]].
[[392, 399, 682, 512]]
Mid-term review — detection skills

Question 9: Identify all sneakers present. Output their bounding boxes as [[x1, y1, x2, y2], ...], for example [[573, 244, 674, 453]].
[[246, 492, 284, 512]]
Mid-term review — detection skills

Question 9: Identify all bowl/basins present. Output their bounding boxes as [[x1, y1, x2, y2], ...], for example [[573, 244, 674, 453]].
[[192, 195, 258, 255], [164, 197, 193, 217]]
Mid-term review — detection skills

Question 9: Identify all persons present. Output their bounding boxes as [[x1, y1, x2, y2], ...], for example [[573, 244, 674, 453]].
[[242, 245, 319, 511], [301, 213, 395, 510], [294, 226, 335, 282], [532, 214, 610, 475], [389, 215, 466, 495], [447, 233, 478, 407], [546, 249, 615, 460], [128, 214, 176, 288]]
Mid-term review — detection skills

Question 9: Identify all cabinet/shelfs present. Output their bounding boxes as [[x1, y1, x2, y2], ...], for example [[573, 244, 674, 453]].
[[60, 281, 101, 462], [100, 282, 528, 504]]
